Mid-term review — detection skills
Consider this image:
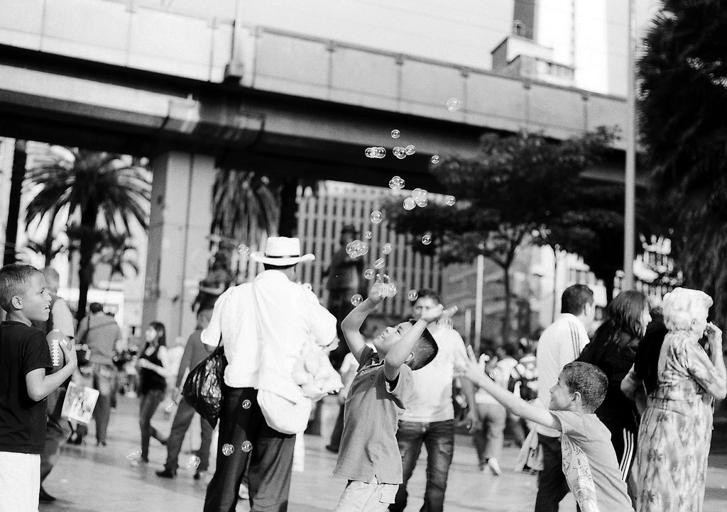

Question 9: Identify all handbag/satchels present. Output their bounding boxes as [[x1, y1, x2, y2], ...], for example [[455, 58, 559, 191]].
[[181, 346, 228, 430]]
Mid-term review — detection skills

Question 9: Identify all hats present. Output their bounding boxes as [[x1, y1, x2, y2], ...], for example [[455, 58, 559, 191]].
[[251, 236, 315, 266]]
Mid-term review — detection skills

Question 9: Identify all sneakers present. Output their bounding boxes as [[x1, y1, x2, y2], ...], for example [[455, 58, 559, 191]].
[[39, 485, 56, 503]]
